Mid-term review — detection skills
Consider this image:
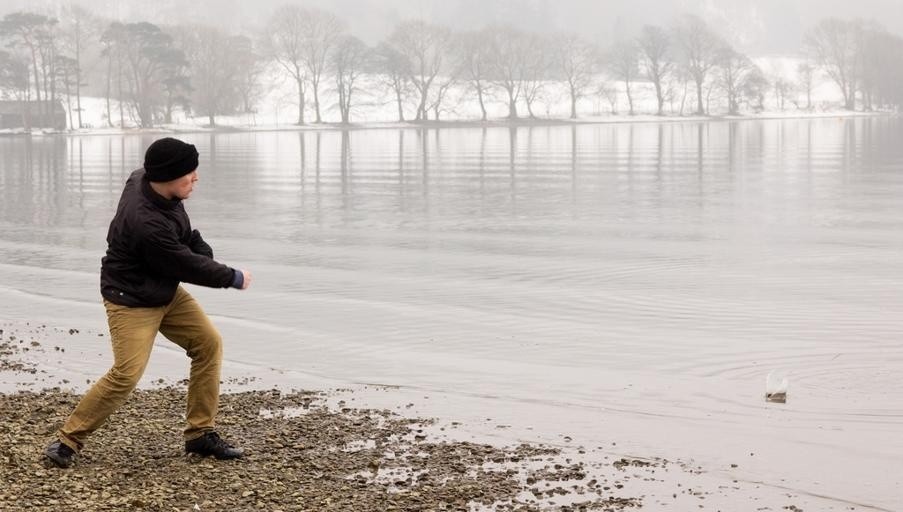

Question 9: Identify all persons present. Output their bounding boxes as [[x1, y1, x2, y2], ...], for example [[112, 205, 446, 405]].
[[45, 137, 251, 468]]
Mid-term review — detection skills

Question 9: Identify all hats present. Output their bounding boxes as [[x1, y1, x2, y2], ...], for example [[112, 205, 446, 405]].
[[144, 138, 198, 183]]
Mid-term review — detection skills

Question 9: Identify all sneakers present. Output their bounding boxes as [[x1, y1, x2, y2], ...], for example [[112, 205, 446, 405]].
[[185, 432, 242, 460], [46, 438, 75, 468]]
